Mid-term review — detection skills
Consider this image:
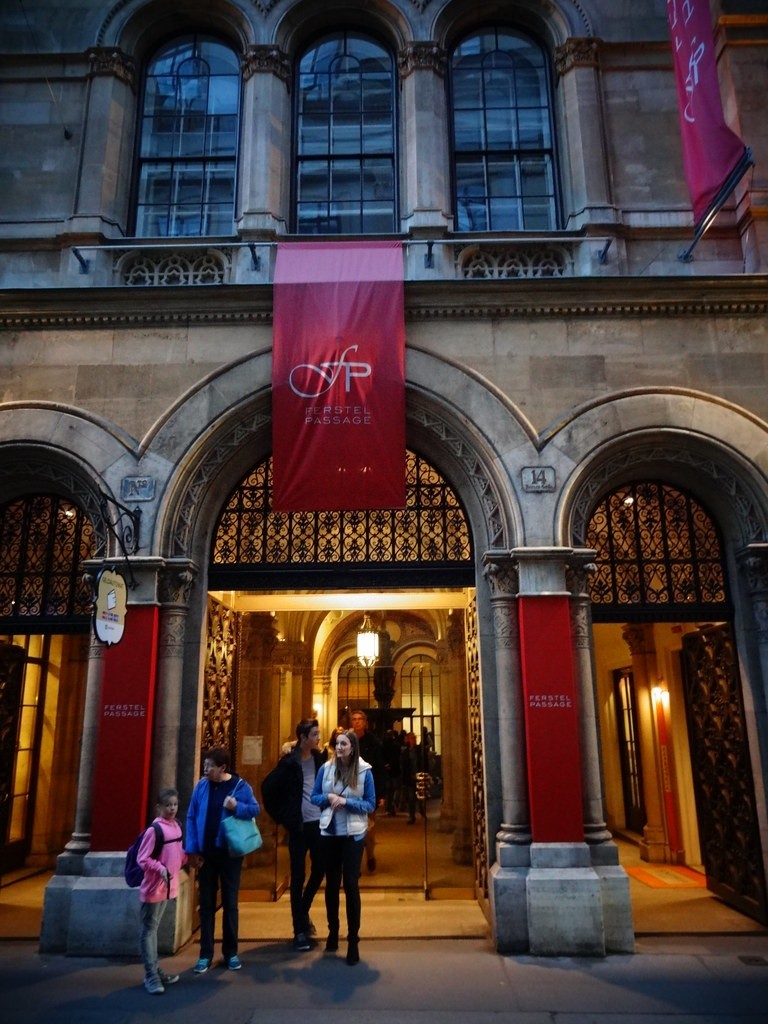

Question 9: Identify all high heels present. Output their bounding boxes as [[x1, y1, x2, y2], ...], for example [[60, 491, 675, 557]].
[[325, 926, 339, 952], [346, 938, 360, 966]]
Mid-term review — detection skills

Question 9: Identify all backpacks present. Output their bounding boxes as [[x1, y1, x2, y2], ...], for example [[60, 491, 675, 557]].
[[124, 817, 185, 888]]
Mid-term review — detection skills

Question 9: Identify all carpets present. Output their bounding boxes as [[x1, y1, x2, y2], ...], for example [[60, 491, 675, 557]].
[[625, 863, 709, 889]]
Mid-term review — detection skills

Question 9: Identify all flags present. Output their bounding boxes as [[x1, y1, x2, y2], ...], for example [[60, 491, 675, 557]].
[[266, 241, 407, 510]]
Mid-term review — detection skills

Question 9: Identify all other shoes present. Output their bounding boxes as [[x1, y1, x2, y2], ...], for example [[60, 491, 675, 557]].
[[307, 918, 317, 935], [294, 934, 310, 950], [366, 856, 376, 872], [418, 807, 425, 816], [406, 816, 416, 825]]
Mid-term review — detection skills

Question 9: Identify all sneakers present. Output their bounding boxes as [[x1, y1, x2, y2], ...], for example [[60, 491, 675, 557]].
[[228, 953, 241, 970], [144, 967, 179, 994], [193, 957, 212, 973]]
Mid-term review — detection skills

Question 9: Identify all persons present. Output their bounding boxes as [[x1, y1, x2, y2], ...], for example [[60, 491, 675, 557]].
[[136, 747, 259, 993], [261, 710, 433, 966]]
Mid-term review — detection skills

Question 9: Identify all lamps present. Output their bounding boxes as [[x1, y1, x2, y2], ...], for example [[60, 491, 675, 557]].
[[357, 611, 380, 670]]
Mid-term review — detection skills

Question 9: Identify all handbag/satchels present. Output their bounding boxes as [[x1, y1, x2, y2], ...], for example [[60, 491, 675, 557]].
[[221, 778, 263, 859]]
